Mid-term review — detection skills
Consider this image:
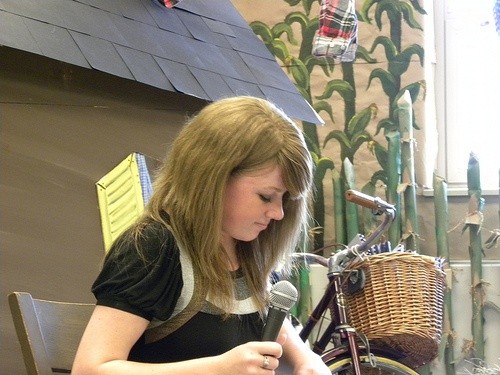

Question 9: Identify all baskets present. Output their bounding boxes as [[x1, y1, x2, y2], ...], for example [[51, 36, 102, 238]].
[[342, 252, 449, 369]]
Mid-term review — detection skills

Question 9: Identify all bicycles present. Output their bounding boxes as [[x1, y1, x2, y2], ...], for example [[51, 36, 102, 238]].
[[277, 189, 449, 372]]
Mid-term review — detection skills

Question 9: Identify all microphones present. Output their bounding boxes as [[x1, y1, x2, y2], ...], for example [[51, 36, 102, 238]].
[[261, 280, 298, 357]]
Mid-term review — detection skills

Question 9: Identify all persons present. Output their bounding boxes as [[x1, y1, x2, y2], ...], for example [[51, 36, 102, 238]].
[[69, 96, 332, 375]]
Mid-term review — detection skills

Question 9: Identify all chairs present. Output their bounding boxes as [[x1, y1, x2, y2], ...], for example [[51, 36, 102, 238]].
[[8, 292, 95, 374]]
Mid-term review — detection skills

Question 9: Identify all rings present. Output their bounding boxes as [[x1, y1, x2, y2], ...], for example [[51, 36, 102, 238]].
[[262, 356, 269, 370]]
